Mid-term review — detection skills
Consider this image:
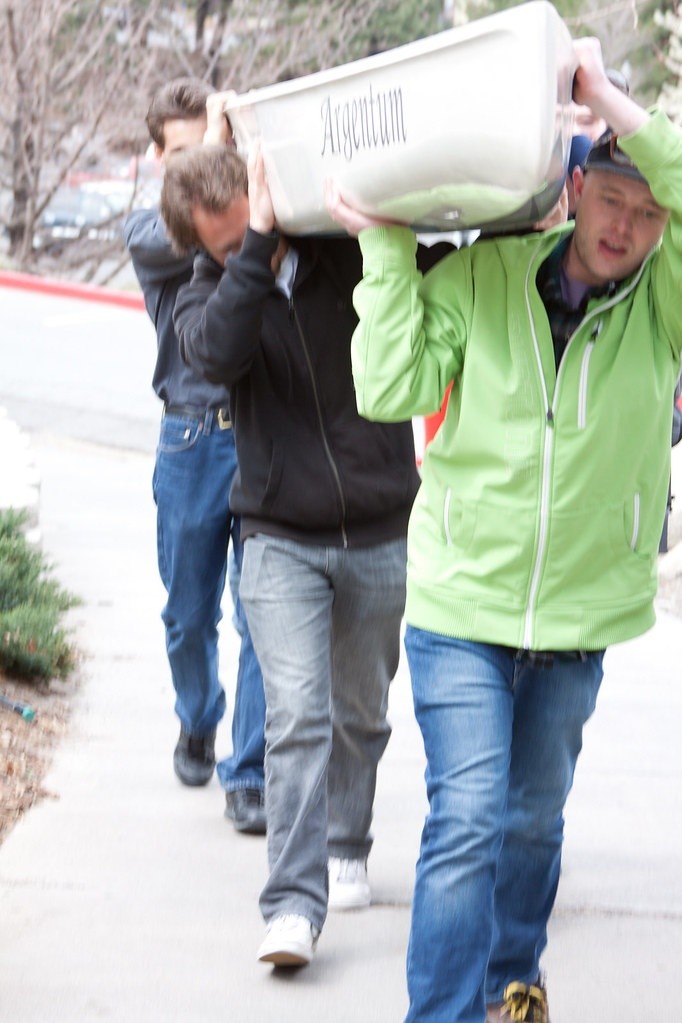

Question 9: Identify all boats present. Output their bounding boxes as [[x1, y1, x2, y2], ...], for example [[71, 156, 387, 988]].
[[220, 1, 586, 240]]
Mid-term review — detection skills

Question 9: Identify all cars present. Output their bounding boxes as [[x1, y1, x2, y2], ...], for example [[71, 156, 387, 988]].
[[0, 139, 171, 254]]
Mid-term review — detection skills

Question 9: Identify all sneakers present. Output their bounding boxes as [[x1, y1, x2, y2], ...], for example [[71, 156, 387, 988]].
[[224, 786, 266, 834], [258, 915, 319, 966], [326, 856, 371, 911], [172, 725, 215, 786]]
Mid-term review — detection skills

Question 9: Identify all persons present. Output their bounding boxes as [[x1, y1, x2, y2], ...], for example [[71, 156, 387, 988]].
[[324, 35, 681, 1022], [129, 75, 262, 835], [161, 142, 569, 966], [480, 68, 681, 511]]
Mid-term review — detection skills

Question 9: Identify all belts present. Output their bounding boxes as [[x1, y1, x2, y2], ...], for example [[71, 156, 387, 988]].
[[164, 404, 232, 430]]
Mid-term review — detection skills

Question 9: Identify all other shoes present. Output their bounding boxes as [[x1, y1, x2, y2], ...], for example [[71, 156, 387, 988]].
[[485, 970, 550, 1023]]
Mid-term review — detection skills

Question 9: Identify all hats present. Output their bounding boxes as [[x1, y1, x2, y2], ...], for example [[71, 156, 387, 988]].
[[583, 127, 652, 183]]
